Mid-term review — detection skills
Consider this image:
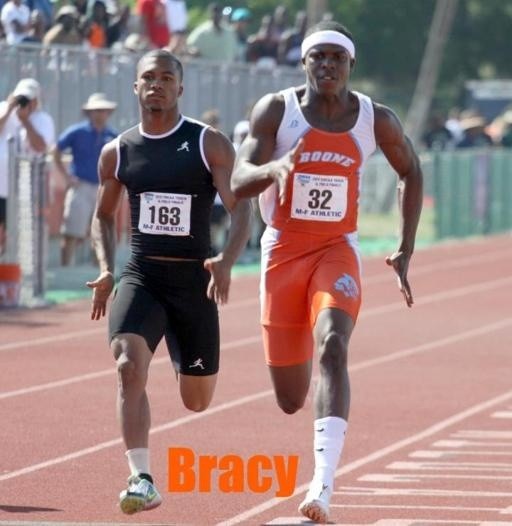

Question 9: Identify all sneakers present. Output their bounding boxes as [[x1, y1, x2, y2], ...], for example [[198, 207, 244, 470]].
[[297, 493, 329, 524], [119, 476, 162, 515]]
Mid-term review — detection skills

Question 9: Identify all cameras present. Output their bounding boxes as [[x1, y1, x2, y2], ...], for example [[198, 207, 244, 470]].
[[17, 97, 29, 108]]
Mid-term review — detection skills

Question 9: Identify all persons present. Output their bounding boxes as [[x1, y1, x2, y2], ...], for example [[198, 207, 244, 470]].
[[84, 47, 258, 517], [411, 105, 511, 231], [230, 19, 424, 525]]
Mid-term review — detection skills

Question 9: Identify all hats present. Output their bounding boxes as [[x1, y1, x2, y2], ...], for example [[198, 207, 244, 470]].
[[13, 78, 41, 101], [230, 8, 253, 22], [81, 92, 119, 110], [56, 4, 79, 23]]
